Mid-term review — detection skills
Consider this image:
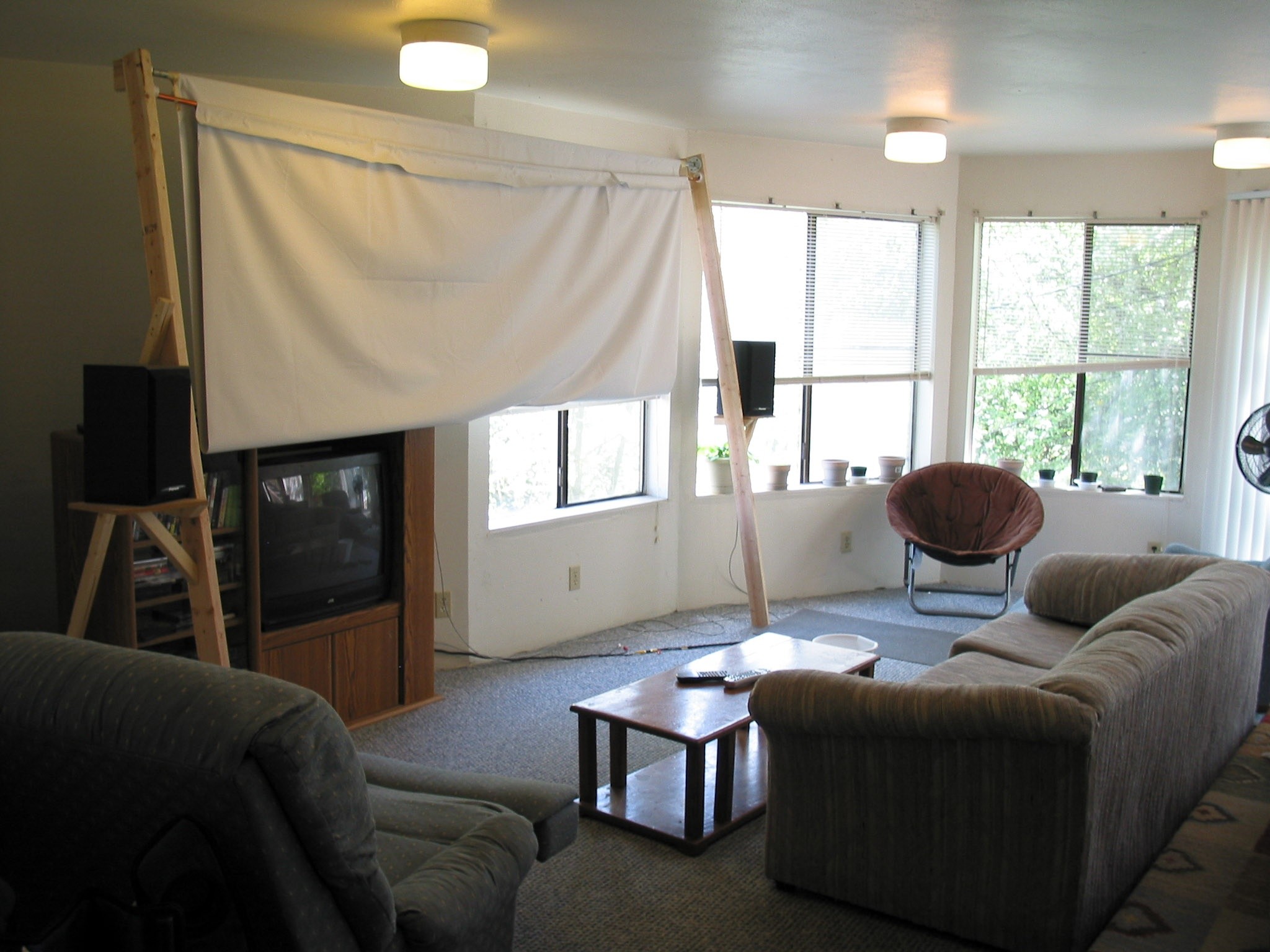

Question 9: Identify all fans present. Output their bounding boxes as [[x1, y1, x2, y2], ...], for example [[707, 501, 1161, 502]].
[[1236, 403, 1270, 494]]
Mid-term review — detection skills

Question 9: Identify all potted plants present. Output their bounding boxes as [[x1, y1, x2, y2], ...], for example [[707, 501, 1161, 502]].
[[697, 443, 759, 493]]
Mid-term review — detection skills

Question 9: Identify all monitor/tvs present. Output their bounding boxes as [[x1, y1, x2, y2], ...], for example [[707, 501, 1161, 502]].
[[197, 430, 399, 629], [82, 364, 196, 505]]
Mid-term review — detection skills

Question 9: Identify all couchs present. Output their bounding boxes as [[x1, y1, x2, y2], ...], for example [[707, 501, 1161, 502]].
[[0, 630, 582, 952], [748, 552, 1270, 952]]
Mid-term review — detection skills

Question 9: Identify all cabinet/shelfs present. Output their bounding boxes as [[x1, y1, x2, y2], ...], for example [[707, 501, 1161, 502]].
[[52, 424, 442, 731], [570, 632, 880, 855]]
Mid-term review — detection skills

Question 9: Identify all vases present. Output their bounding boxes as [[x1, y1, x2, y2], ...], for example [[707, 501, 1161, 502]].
[[1144, 474, 1163, 495], [823, 459, 866, 482], [1040, 470, 1056, 479], [997, 458, 1024, 477], [767, 464, 791, 490], [1080, 472, 1097, 482], [878, 456, 905, 478]]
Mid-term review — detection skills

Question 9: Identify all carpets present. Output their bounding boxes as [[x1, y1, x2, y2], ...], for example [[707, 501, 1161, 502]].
[[510, 608, 1269, 951]]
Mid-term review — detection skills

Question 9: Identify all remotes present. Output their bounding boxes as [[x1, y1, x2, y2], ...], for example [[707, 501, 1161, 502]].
[[723, 668, 772, 689], [676, 670, 730, 683]]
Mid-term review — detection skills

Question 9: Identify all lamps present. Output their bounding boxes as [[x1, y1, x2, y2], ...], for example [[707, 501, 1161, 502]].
[[398, 19, 493, 92], [884, 117, 951, 163], [1212, 122, 1270, 170]]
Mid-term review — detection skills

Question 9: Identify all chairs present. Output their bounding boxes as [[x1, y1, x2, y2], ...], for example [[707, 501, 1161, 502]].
[[886, 461, 1044, 618]]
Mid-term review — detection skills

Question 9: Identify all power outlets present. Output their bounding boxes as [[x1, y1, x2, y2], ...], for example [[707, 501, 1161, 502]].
[[1146, 541, 1162, 554], [570, 566, 581, 590], [839, 530, 852, 554], [436, 590, 450, 618]]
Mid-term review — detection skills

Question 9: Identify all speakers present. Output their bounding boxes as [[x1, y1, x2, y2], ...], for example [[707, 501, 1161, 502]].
[[717, 341, 774, 418]]
